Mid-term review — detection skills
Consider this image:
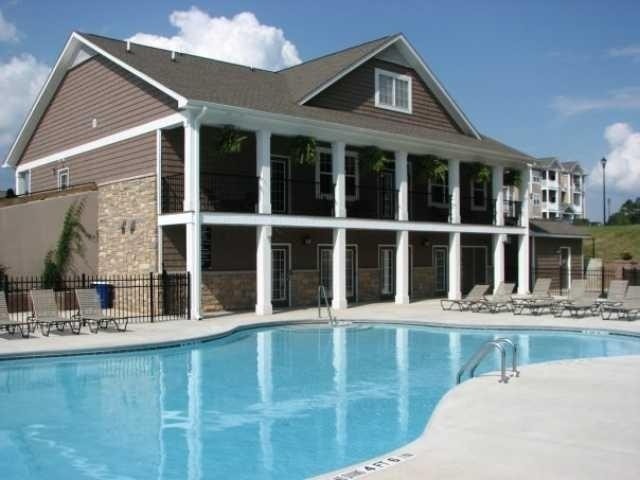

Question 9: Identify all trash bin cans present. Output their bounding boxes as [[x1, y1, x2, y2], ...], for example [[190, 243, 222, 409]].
[[90, 282, 113, 309]]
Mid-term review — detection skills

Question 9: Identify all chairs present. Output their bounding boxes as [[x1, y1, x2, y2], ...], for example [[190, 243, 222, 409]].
[[440, 277, 640, 321], [0, 288, 128, 338]]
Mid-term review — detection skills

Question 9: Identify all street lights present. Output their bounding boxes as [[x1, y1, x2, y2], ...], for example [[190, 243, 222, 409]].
[[600, 157, 607, 225]]
